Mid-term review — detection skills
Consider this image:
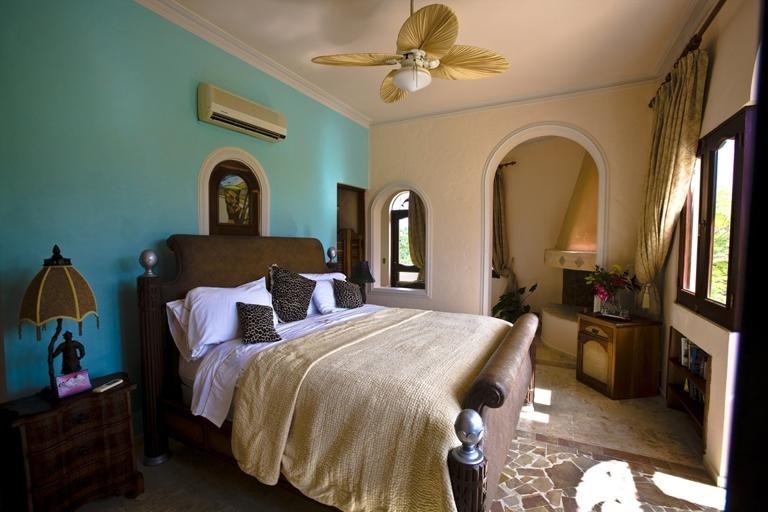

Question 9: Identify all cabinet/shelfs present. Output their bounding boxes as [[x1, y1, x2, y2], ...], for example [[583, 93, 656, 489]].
[[576, 312, 664, 400], [666, 358, 706, 430]]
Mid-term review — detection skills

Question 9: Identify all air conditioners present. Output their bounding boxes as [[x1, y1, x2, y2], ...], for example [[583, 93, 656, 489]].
[[195, 81, 288, 145]]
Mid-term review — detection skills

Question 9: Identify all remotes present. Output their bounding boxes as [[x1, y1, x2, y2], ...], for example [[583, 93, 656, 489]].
[[92, 378, 124, 394]]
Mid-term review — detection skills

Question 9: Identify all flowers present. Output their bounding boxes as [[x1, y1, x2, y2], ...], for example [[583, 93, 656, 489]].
[[583, 264, 643, 303]]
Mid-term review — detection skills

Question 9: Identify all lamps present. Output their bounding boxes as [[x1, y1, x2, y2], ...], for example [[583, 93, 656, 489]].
[[17, 244, 100, 399], [308, 1, 509, 105]]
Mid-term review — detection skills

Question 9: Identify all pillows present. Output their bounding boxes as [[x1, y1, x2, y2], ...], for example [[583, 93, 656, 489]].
[[299, 269, 344, 316], [309, 273, 333, 315], [166, 275, 269, 363], [235, 298, 280, 345], [334, 275, 363, 309], [272, 265, 316, 323], [182, 286, 278, 357]]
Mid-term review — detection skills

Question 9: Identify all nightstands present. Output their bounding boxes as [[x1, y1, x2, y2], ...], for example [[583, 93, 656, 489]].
[[1, 373, 144, 512]]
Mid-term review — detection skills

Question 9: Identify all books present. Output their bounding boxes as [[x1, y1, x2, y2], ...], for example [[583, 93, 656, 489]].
[[681, 338, 708, 405]]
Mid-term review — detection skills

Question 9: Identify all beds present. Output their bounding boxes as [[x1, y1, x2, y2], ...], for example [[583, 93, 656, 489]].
[[136, 235, 540, 512]]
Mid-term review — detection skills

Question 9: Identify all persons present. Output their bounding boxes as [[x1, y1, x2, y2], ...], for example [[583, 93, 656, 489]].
[[52, 332, 85, 374]]
